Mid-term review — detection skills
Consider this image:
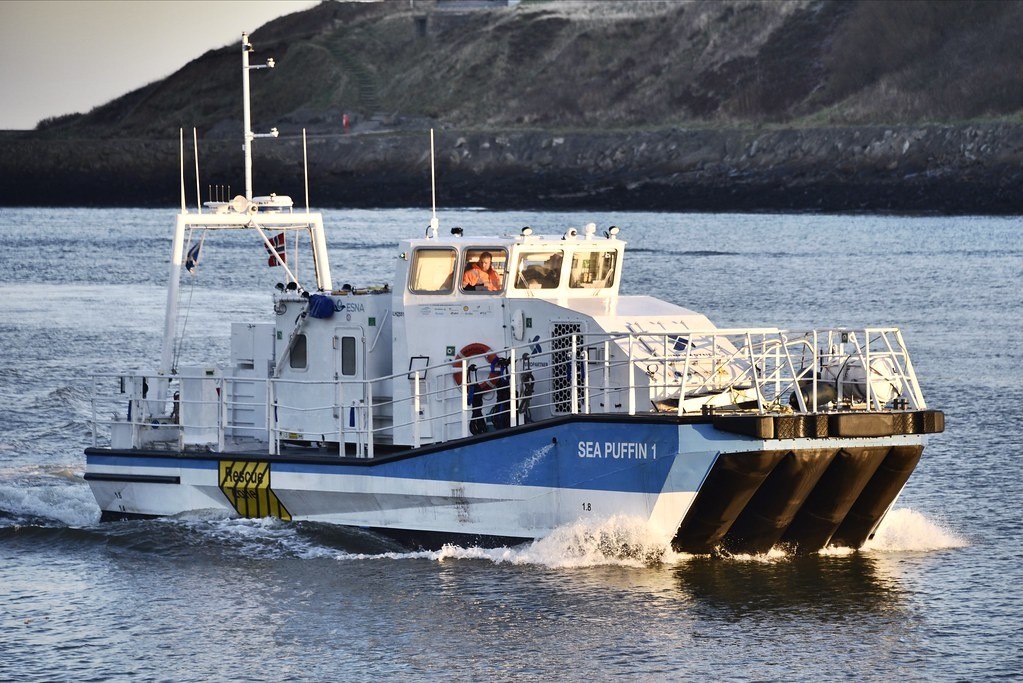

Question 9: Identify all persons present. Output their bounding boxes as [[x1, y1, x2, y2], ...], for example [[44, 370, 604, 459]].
[[461, 251, 502, 290]]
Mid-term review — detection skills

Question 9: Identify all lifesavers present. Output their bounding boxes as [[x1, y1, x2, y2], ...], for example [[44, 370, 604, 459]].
[[454, 343, 502, 391]]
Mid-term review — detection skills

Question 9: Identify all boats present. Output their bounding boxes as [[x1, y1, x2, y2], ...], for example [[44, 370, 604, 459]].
[[78, 26, 956, 571]]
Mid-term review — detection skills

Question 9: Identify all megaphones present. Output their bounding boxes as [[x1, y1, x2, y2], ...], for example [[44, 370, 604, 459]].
[[232, 195, 248, 212]]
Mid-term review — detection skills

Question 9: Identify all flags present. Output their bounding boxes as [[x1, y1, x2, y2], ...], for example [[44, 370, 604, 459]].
[[185, 242, 200, 272], [264, 232, 285, 266]]
